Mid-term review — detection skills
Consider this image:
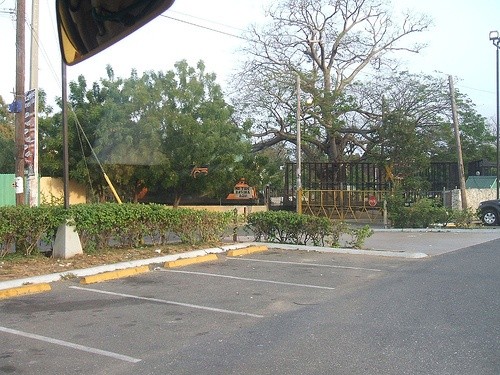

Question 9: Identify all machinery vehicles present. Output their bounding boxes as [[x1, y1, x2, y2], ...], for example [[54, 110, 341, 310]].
[[191, 165, 261, 206]]
